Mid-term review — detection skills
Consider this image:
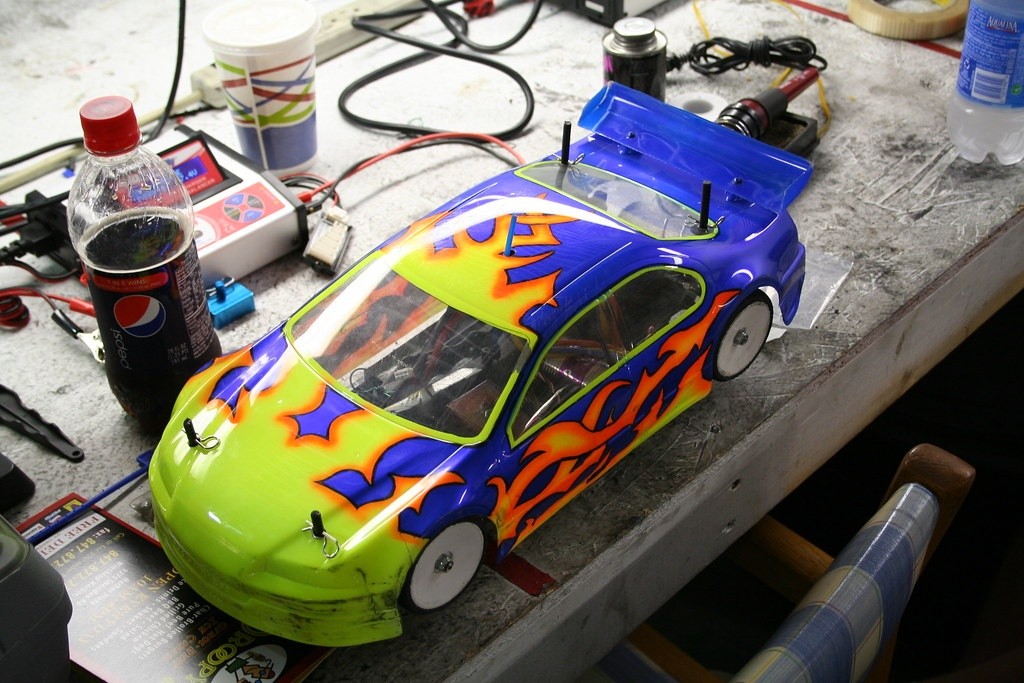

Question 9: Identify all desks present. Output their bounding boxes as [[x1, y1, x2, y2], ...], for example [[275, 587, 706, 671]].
[[0, 0, 1024, 683]]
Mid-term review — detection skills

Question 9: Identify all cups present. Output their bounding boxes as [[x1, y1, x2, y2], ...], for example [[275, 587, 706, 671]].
[[201, 0, 323, 179]]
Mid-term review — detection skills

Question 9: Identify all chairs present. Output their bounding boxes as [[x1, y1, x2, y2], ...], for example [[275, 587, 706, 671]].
[[575, 444, 976, 683]]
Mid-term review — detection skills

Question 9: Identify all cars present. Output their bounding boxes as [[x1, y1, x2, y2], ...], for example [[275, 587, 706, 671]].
[[144, 80, 818, 649]]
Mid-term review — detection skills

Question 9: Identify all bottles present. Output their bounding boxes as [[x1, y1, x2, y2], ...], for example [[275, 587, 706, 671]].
[[65, 96, 224, 435], [945, 0, 1024, 167], [601, 16, 667, 104]]
